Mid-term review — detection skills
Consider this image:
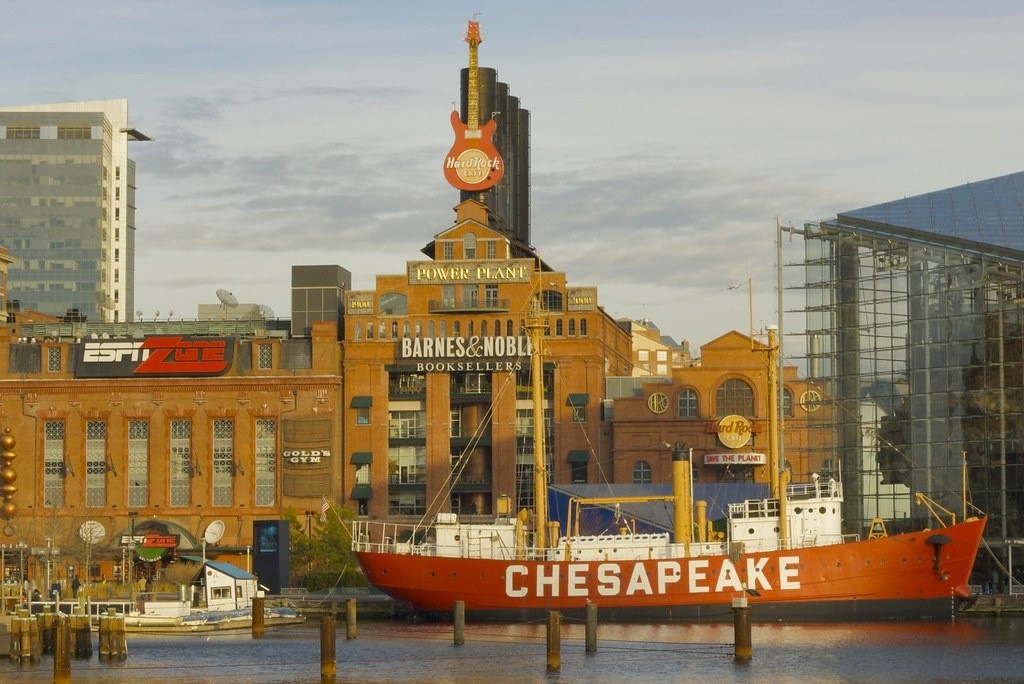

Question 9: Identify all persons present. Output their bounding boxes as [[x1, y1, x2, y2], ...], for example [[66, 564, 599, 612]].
[[71, 573, 81, 599], [138, 576, 152, 600], [31, 589, 40, 601], [991, 570, 1000, 593]]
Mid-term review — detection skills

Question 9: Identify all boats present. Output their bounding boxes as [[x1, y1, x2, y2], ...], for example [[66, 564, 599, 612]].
[[28, 560, 305, 631], [351, 249, 990, 616]]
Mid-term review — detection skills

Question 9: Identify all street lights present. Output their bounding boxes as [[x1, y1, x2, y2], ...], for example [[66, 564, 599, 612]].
[[17, 535, 25, 606], [44, 537, 53, 595], [128, 510, 139, 541]]
